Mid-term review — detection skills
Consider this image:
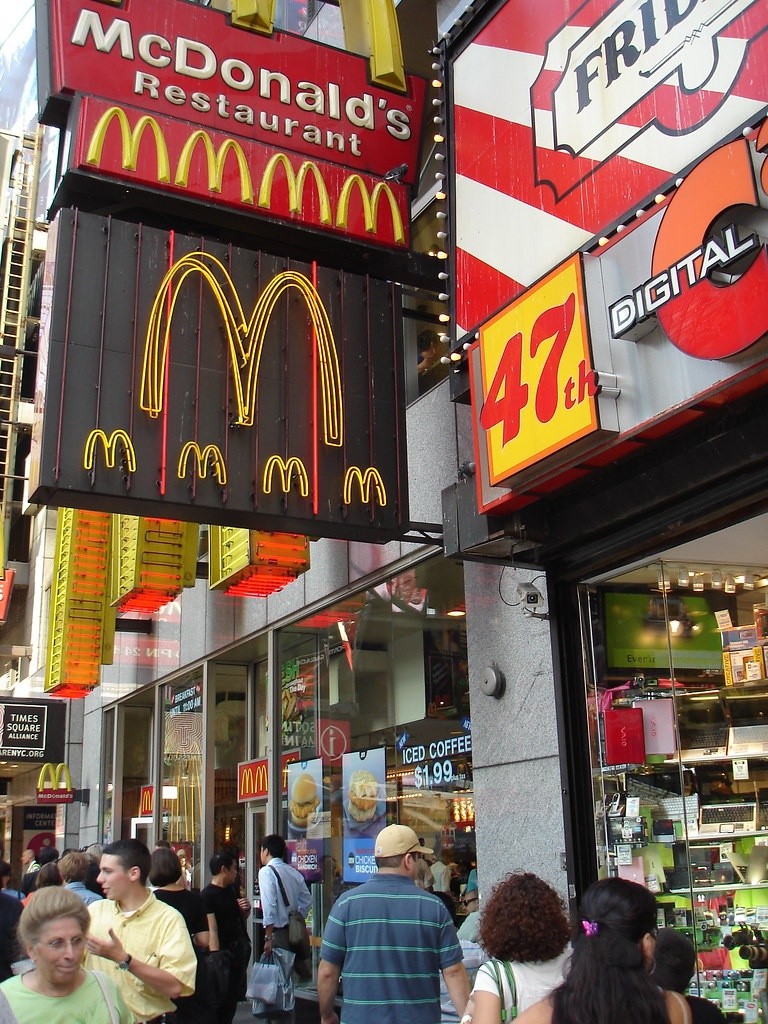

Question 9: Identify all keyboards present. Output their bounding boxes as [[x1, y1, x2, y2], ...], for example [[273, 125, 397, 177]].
[[699, 802, 757, 833], [660, 794, 699, 823]]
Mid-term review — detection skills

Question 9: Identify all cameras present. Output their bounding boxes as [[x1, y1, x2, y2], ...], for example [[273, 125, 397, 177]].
[[704, 969, 753, 992]]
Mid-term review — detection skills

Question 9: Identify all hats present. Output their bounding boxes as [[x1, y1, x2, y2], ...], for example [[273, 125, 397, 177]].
[[375, 824, 434, 857]]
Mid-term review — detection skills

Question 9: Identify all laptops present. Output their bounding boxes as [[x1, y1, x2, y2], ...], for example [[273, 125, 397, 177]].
[[673, 689, 728, 759], [603, 707, 647, 764], [723, 684, 768, 756], [633, 697, 676, 755]]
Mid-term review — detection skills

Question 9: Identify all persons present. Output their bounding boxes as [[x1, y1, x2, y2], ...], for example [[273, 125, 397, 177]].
[[317, 824, 472, 1024], [460, 868, 727, 1024], [419, 839, 479, 932], [0, 841, 251, 1024], [258, 834, 312, 1024]]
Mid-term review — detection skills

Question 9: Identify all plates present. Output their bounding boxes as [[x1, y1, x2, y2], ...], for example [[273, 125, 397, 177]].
[[287, 810, 322, 832]]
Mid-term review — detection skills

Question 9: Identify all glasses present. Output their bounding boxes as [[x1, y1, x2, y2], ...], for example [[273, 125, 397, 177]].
[[648, 925, 658, 937], [464, 898, 478, 906], [34, 936, 88, 951], [406, 838, 424, 854]]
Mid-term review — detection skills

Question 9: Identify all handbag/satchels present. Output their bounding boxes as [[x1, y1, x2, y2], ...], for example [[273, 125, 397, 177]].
[[252, 951, 295, 1019], [288, 911, 306, 945], [244, 953, 279, 1004]]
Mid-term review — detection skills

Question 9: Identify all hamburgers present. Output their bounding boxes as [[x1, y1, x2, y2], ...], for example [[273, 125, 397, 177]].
[[290, 773, 320, 826], [349, 770, 378, 822]]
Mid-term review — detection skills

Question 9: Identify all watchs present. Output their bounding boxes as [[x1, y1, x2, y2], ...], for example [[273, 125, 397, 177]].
[[119, 954, 132, 971]]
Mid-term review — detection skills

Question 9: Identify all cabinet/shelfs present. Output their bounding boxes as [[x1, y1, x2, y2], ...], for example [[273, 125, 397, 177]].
[[652, 833, 768, 897]]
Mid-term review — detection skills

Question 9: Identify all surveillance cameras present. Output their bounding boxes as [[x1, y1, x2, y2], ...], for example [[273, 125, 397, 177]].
[[516, 583, 545, 607]]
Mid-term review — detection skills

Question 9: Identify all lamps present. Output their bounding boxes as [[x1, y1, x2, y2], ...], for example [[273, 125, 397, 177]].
[[742, 576, 754, 591], [657, 569, 671, 590], [725, 577, 736, 594], [711, 572, 723, 589], [676, 567, 689, 587], [692, 576, 704, 591]]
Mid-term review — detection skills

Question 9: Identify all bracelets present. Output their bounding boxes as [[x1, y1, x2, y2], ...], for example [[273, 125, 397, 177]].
[[461, 1014, 473, 1024], [265, 934, 272, 941]]
